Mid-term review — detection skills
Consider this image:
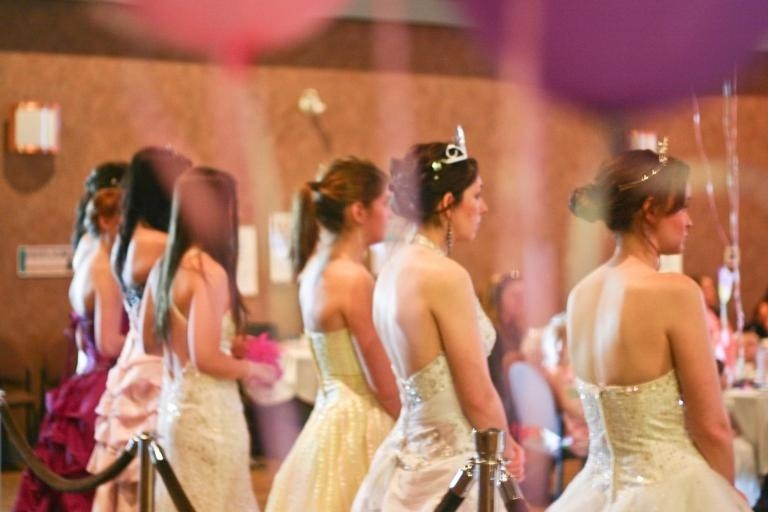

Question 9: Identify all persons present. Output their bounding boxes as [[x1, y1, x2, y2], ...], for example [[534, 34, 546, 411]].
[[141, 167, 276, 512], [263, 159, 403, 512], [350, 143, 527, 512], [68, 163, 125, 376], [487, 274, 587, 426], [690, 275, 768, 390], [544, 150, 752, 512], [86, 146, 192, 512], [13, 188, 130, 512]]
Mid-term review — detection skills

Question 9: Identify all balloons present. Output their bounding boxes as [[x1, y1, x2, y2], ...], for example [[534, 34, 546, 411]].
[[89, 0, 351, 81], [452, 0, 768, 117]]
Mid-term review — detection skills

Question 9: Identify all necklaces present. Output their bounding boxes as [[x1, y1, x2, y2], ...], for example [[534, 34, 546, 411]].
[[408, 232, 444, 256]]
